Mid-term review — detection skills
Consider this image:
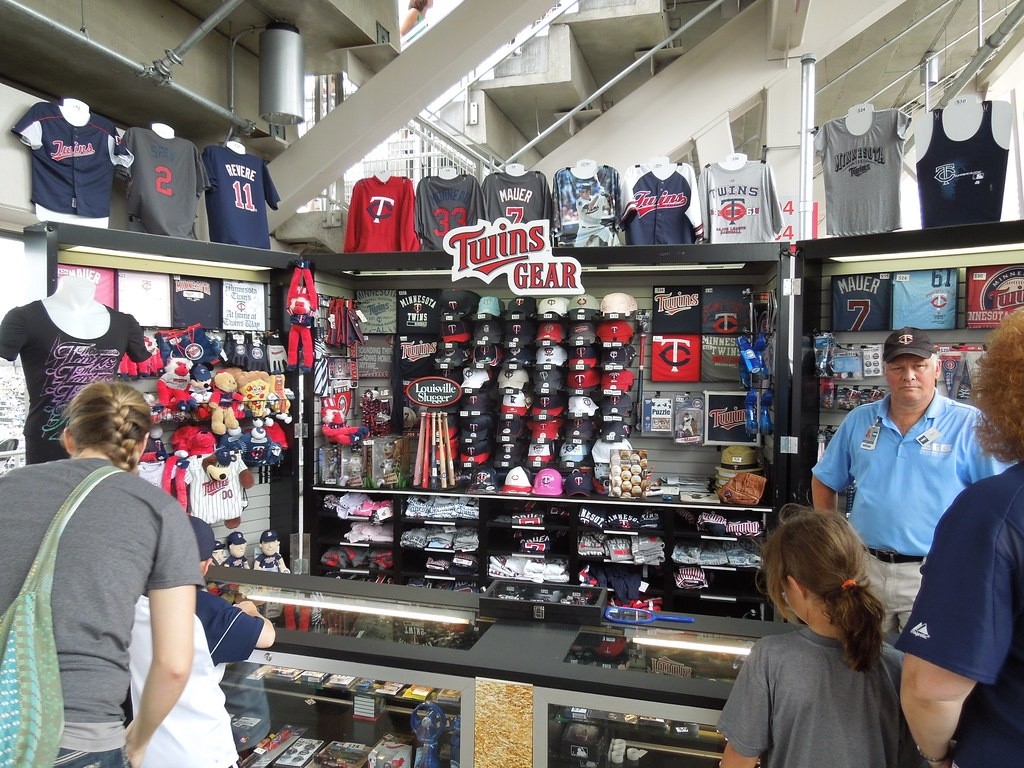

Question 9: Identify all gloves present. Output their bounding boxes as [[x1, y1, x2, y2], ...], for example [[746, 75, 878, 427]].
[[223, 332, 288, 374]]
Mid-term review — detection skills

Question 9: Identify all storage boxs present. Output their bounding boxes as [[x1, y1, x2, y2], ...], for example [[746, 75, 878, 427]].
[[479, 580, 608, 626], [368, 740, 413, 768]]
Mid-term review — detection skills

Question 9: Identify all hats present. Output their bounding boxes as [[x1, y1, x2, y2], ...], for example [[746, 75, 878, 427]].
[[883, 326, 937, 361], [715, 444, 765, 489], [193, 364, 208, 384], [185, 515, 216, 563], [430, 292, 638, 499]]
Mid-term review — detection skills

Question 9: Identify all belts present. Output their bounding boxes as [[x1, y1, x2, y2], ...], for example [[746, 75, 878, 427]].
[[867, 547, 922, 563]]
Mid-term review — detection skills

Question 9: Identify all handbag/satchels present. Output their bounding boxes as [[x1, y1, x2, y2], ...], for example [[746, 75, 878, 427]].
[[0, 593, 66, 768]]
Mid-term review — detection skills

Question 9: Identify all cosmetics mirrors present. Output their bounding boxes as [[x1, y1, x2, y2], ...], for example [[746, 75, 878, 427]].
[[605, 607, 695, 624]]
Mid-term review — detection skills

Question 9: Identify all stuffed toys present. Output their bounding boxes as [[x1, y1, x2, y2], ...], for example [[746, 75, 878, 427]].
[[212, 530, 291, 574], [189, 362, 213, 403], [157, 357, 198, 412], [139, 425, 169, 464], [287, 259, 317, 373], [171, 423, 216, 459], [208, 367, 294, 436], [320, 398, 368, 445], [162, 455, 187, 511], [218, 428, 283, 467], [184, 446, 255, 529]]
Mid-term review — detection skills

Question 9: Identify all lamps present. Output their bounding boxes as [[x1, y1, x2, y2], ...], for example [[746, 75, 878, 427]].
[[624, 628, 755, 655], [920, 51, 938, 87], [239, 587, 476, 626], [259, 21, 305, 126]]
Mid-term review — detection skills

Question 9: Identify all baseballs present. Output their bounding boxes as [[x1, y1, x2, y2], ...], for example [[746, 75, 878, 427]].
[[608, 448, 648, 499]]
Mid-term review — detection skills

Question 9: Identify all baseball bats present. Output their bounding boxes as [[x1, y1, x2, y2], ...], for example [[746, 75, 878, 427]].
[[442, 412, 455, 486], [431, 412, 437, 490], [413, 412, 426, 485], [436, 412, 448, 489], [422, 413, 431, 489]]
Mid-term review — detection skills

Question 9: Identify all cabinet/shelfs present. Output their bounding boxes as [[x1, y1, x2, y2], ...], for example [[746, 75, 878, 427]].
[[310, 493, 774, 623]]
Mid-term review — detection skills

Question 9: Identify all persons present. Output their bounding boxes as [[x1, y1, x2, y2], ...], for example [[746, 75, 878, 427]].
[[715, 486, 929, 768], [120, 515, 276, 768], [0, 379, 208, 768], [812, 327, 1018, 632], [0, 275, 152, 466], [893, 305, 1024, 768]]
